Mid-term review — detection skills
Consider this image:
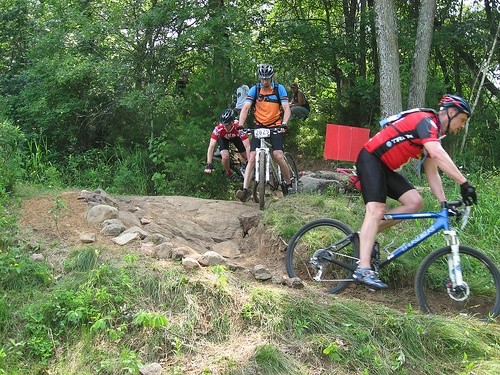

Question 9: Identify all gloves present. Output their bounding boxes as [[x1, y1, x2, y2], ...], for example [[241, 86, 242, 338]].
[[460, 181, 478, 206], [446, 200, 462, 216]]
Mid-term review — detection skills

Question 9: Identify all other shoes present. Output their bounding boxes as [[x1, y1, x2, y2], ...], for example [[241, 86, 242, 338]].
[[287, 183, 293, 195], [371, 243, 380, 263], [352, 267, 388, 289], [235, 190, 246, 202]]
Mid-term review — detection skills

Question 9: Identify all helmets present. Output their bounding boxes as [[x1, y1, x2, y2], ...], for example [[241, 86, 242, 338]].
[[220, 108, 235, 125], [291, 83, 298, 88], [257, 63, 275, 79], [438, 92, 471, 117]]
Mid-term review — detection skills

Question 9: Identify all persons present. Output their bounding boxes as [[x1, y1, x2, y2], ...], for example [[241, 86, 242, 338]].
[[204, 64, 310, 203], [353, 94, 477, 288], [176, 69, 203, 114]]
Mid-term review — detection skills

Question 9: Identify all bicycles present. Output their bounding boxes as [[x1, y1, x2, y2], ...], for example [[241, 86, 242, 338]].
[[241, 125, 289, 209], [230, 94, 238, 109], [286, 188, 500, 323], [253, 150, 299, 210], [208, 144, 281, 204]]
